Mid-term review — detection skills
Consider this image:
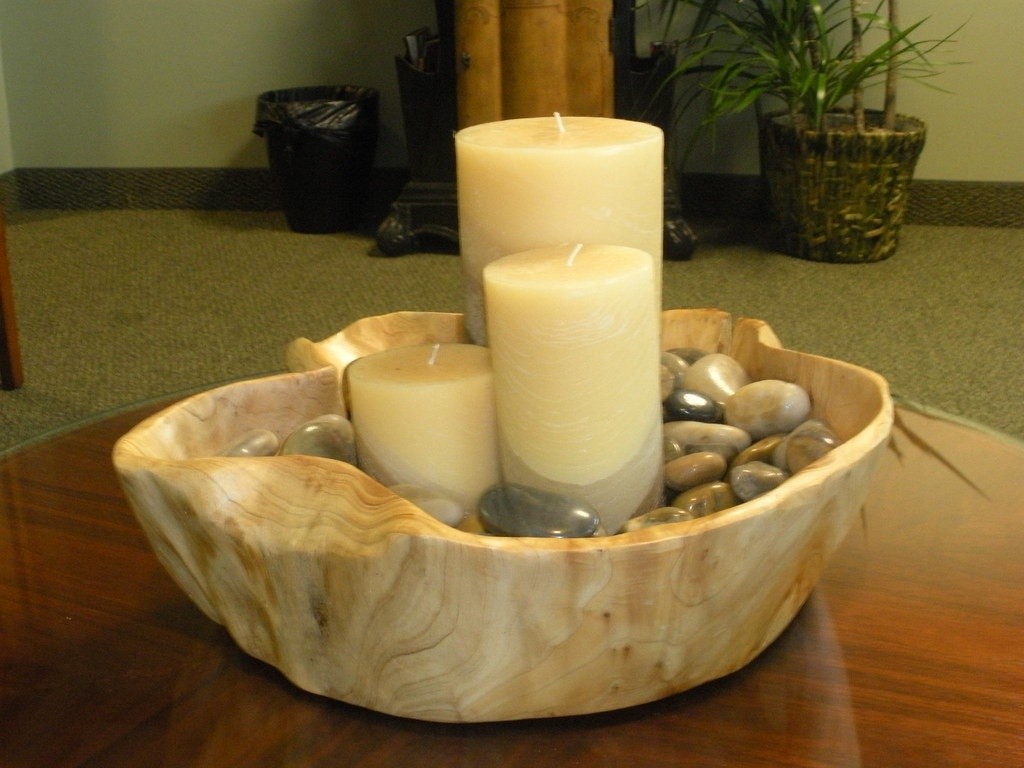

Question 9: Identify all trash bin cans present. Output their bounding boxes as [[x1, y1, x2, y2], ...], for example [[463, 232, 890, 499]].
[[257, 84, 390, 235]]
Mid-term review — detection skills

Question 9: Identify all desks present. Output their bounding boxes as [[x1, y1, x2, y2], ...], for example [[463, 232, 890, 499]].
[[0, 368, 1023, 768]]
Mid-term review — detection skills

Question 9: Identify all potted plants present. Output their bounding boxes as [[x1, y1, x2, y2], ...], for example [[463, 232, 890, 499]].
[[623, 1, 973, 264]]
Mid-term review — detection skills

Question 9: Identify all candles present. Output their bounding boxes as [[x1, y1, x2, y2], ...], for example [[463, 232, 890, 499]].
[[455, 112, 664, 350], [346, 343, 497, 510], [483, 243, 664, 537]]
